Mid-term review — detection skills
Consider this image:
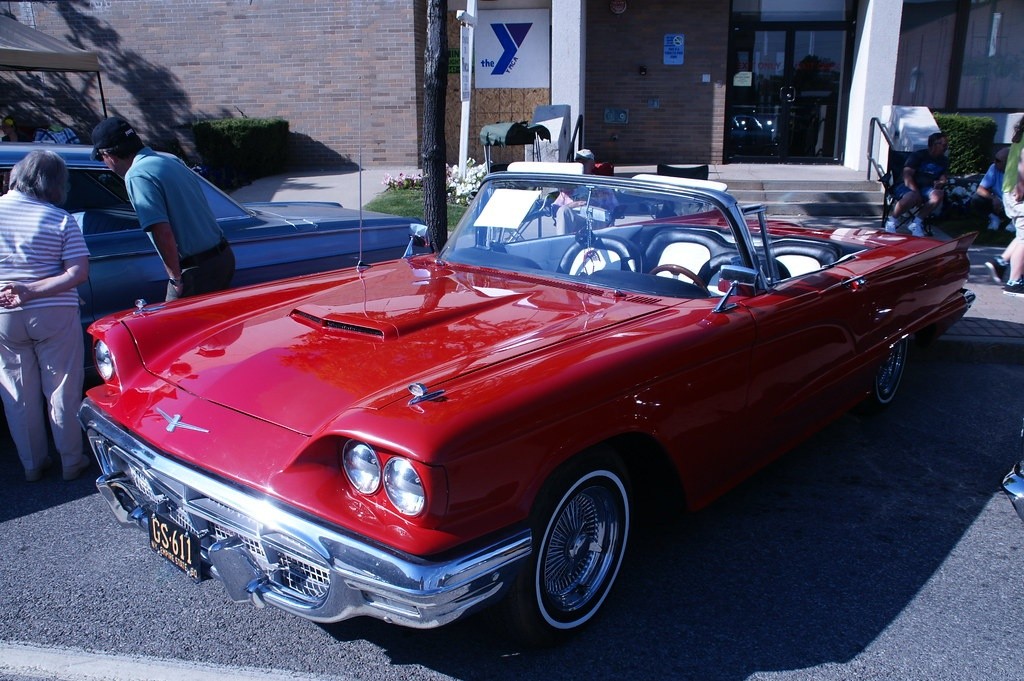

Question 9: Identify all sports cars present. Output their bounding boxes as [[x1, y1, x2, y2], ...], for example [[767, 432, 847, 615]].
[[75, 159, 981, 653]]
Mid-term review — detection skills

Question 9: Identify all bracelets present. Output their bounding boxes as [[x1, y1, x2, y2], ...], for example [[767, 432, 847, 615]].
[[991, 192, 996, 199]]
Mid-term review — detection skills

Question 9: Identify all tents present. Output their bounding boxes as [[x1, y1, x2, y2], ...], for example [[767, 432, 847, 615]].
[[0, 15, 108, 121]]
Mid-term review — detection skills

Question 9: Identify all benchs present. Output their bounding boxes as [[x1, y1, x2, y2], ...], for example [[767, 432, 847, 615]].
[[646, 228, 838, 290]]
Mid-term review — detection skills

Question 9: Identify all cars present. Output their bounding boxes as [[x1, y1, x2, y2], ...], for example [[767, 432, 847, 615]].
[[731, 71, 797, 160], [0, 141, 418, 437]]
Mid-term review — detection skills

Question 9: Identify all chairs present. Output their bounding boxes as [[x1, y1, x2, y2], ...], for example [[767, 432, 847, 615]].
[[557, 233, 642, 278], [882, 146, 936, 237], [695, 250, 792, 290]]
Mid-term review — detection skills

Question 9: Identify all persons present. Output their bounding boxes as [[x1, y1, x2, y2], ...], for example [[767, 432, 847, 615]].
[[974, 145, 1016, 232], [90, 117, 237, 303], [551, 148, 618, 236], [1, 115, 28, 141], [0, 151, 91, 483], [885, 133, 949, 238], [984, 114, 1024, 297]]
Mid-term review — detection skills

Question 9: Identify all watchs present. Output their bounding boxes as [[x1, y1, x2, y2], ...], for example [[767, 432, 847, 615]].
[[169, 277, 182, 286]]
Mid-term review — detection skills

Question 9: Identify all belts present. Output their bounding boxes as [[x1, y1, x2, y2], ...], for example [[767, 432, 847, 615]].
[[179, 237, 228, 268]]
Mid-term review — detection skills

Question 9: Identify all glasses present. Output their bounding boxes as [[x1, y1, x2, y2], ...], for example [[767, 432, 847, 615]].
[[994, 157, 1005, 163], [95, 150, 111, 163], [935, 143, 946, 148]]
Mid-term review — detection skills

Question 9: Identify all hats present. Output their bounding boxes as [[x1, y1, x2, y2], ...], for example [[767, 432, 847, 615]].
[[90, 117, 136, 160], [574, 149, 594, 160]]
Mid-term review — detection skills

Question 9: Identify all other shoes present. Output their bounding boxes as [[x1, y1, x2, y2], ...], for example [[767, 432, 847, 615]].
[[25, 456, 48, 482], [63, 454, 90, 480], [985, 259, 1007, 283], [1005, 275, 1024, 294]]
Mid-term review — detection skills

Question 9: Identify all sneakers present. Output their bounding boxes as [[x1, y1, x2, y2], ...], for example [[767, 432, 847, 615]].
[[908, 221, 924, 237], [885, 217, 895, 233]]
